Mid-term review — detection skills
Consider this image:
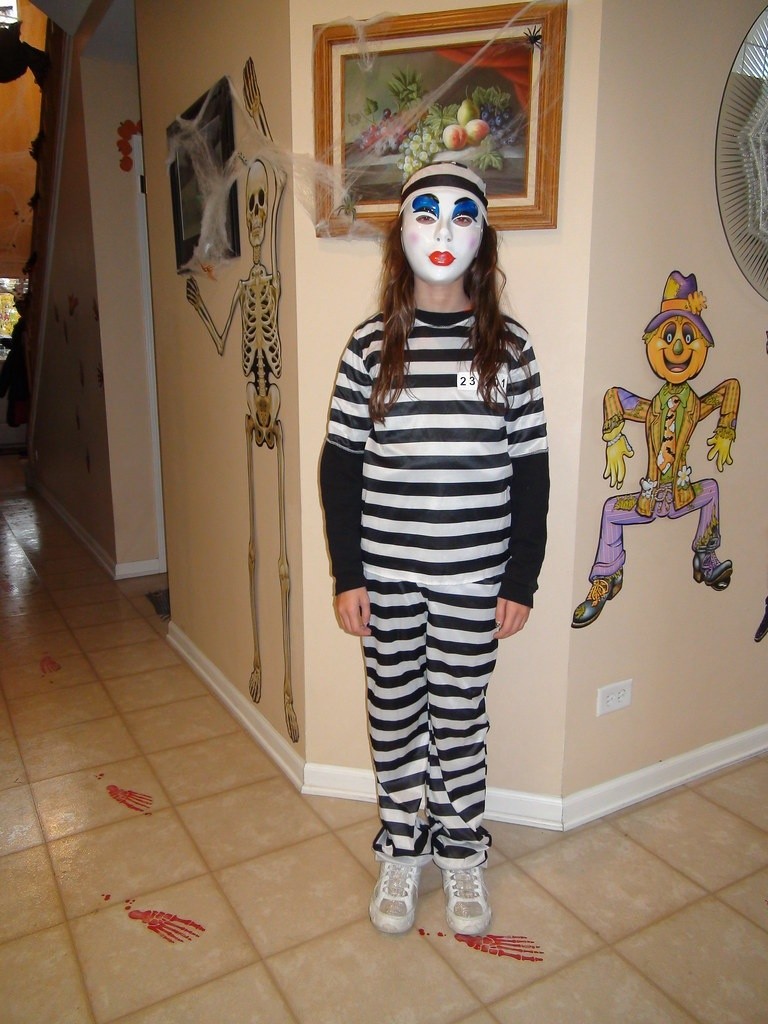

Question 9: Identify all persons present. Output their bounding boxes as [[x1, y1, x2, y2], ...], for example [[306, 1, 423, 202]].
[[319, 159, 552, 932]]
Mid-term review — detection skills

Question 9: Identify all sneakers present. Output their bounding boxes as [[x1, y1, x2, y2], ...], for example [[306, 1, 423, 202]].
[[370, 861, 423, 933], [441, 866, 491, 935]]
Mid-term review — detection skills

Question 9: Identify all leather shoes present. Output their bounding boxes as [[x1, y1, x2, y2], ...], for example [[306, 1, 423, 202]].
[[571, 567, 623, 628], [693, 552, 733, 587]]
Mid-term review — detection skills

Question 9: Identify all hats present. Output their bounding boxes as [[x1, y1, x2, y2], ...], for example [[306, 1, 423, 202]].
[[644, 270, 714, 348]]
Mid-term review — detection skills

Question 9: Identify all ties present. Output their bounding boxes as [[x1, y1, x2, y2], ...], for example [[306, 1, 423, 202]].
[[655, 395, 680, 475]]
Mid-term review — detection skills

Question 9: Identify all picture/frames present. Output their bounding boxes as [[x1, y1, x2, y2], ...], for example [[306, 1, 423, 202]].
[[165, 76, 241, 274], [313, 0, 568, 238]]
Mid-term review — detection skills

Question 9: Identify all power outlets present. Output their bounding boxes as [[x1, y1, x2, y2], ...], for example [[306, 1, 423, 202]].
[[596, 678, 632, 717]]
